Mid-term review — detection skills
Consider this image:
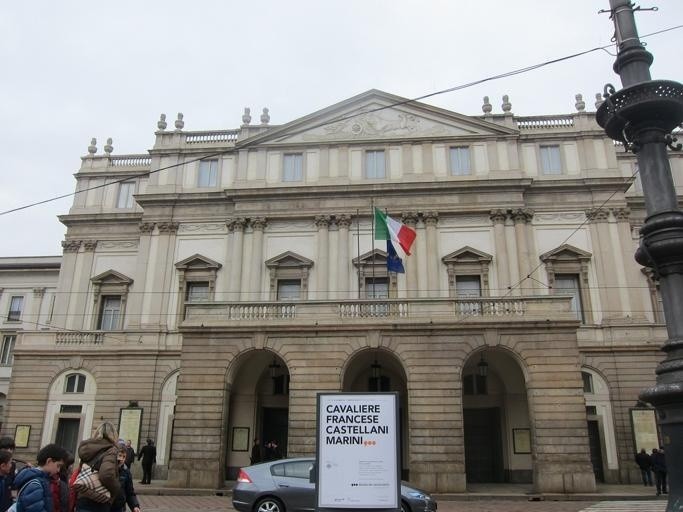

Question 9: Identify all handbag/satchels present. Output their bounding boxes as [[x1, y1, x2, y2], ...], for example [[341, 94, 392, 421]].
[[73, 446, 115, 504]]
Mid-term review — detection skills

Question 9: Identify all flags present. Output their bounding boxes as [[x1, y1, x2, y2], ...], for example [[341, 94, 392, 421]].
[[387, 228, 405, 273], [374, 206, 416, 256]]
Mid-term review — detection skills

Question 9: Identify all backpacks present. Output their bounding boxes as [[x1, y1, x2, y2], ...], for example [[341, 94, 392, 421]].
[[5, 479, 44, 512]]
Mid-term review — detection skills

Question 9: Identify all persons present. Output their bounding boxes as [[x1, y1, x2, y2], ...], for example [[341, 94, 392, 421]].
[[0, 421, 156, 512], [251, 437, 287, 466], [636, 446, 669, 496]]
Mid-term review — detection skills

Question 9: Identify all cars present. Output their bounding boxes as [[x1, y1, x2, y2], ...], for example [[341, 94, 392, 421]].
[[230, 457, 439, 512]]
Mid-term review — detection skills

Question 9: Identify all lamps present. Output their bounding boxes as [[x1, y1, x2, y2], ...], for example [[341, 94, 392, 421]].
[[477, 351, 488, 377], [369, 351, 380, 378], [268, 354, 280, 377]]
